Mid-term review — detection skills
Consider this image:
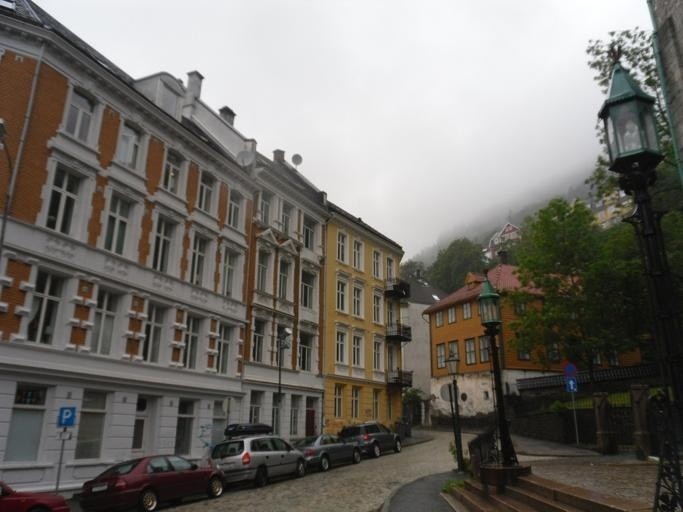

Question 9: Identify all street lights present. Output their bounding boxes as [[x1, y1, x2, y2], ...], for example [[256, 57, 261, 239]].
[[275, 324, 294, 404], [475, 262, 521, 466], [442, 348, 466, 473], [594, 40, 682, 512]]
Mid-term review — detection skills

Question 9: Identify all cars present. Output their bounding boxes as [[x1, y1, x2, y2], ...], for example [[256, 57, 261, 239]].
[[289, 431, 362, 474], [0, 478, 68, 511], [79, 454, 229, 511]]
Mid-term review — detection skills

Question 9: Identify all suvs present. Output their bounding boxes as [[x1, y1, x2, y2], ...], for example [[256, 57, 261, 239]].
[[336, 420, 403, 460], [207, 421, 307, 490]]
